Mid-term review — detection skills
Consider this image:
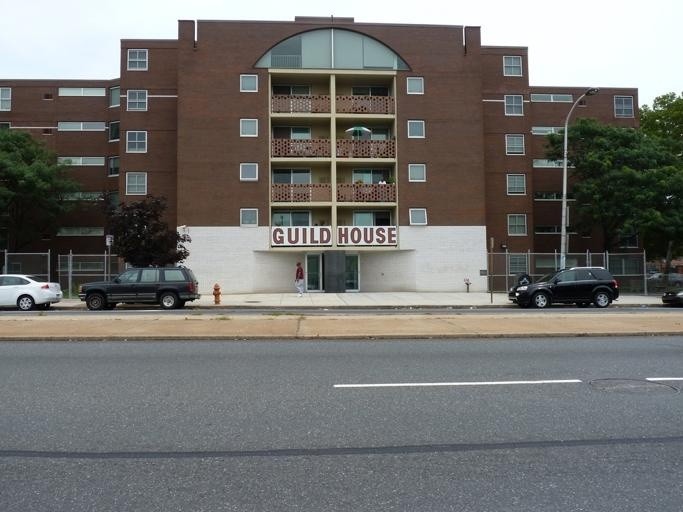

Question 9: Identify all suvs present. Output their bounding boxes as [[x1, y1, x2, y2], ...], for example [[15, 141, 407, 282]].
[[78, 265, 200, 310]]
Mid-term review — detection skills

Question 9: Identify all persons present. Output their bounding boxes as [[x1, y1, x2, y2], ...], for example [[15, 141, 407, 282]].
[[295, 262, 305, 297], [379, 176, 387, 184]]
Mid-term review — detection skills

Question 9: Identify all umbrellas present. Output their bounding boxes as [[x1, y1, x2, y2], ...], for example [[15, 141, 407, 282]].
[[345, 126, 372, 140]]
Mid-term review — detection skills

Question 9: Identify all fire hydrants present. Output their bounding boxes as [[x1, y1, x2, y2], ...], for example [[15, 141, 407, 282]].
[[212, 283, 221, 304]]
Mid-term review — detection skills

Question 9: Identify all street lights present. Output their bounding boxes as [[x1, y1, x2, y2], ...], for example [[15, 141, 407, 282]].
[[560, 87, 600, 271]]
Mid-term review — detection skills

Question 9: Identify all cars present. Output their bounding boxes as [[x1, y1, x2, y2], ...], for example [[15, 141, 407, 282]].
[[648, 272, 683, 287], [0, 274, 63, 310], [662, 289, 683, 307], [508, 266, 619, 307]]
